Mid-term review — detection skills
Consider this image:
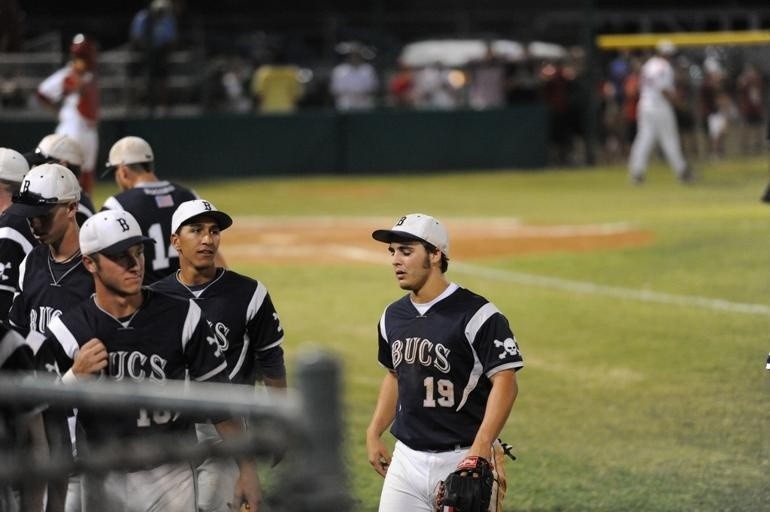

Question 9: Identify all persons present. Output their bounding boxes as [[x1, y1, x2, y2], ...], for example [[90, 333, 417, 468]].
[[516, 37, 770, 183], [195, 51, 510, 122], [2, 136, 290, 511], [364, 213, 526, 512], [34, 23, 102, 188], [124, 4, 188, 115]]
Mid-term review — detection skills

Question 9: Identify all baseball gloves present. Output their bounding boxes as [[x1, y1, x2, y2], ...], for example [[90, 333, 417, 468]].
[[437, 458, 492, 510]]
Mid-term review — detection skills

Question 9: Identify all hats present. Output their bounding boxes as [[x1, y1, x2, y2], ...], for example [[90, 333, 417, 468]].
[[21, 131, 85, 167], [78, 209, 156, 259], [0, 146, 31, 187], [170, 198, 234, 237], [99, 136, 154, 182], [2, 163, 84, 218], [370, 214, 451, 262]]
